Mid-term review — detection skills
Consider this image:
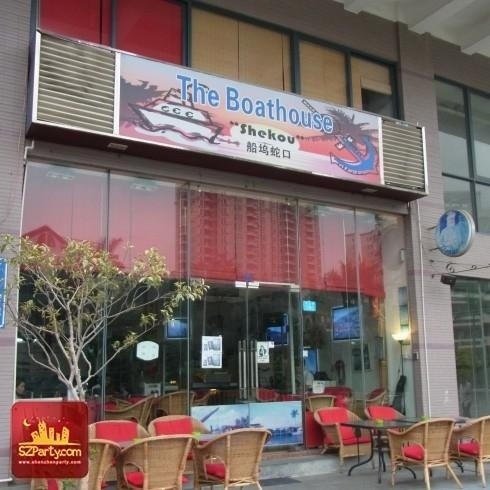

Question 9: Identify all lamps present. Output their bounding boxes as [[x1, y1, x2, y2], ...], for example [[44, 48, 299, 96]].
[[136, 341, 159, 361], [391, 334, 408, 415]]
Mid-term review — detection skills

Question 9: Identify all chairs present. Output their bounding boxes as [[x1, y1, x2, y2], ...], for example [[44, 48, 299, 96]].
[[317, 405, 490, 489], [31, 389, 273, 490], [254, 386, 388, 451]]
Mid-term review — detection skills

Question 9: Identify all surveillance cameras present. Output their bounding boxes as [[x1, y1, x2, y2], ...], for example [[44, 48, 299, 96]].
[[441, 274, 457, 285]]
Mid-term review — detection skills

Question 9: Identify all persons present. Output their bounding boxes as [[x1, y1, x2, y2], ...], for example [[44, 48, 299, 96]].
[[295, 357, 316, 392], [16, 376, 26, 399]]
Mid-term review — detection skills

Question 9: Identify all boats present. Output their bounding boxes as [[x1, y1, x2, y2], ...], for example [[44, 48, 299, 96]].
[[124, 86, 222, 144]]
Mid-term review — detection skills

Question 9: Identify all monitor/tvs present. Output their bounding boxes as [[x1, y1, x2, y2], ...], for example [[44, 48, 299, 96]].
[[265, 313, 288, 347], [165, 316, 192, 341], [302, 299, 318, 313], [331, 305, 363, 343], [304, 347, 320, 379]]
